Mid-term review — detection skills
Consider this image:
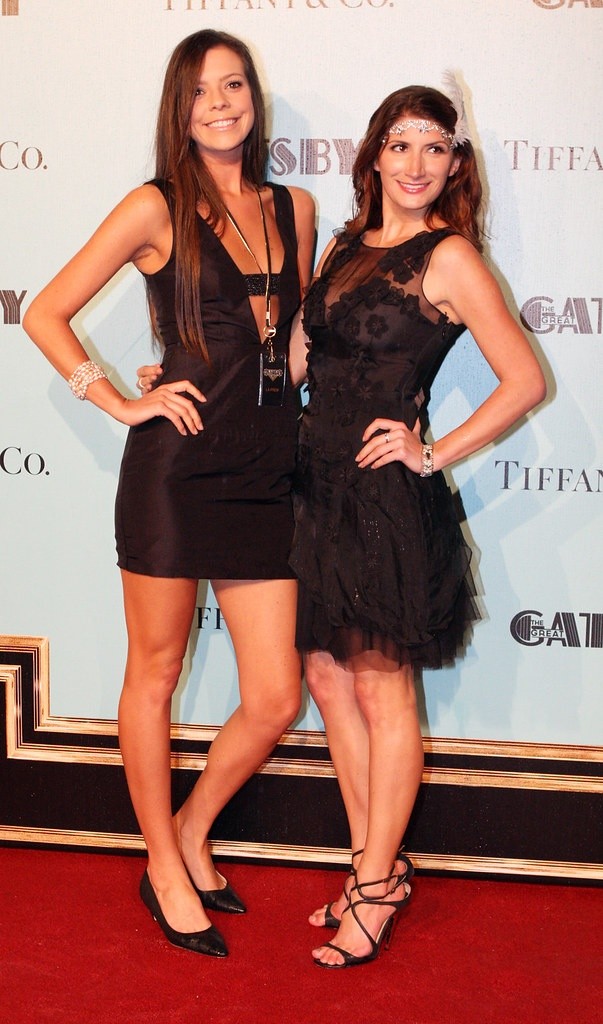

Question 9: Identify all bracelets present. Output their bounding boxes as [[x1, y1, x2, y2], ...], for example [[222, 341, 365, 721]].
[[69, 361, 107, 401], [419, 443, 434, 479]]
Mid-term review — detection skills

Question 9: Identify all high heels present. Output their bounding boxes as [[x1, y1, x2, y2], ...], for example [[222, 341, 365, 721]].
[[320, 848, 414, 927], [314, 872, 411, 968], [140, 865, 229, 960], [184, 859, 247, 914]]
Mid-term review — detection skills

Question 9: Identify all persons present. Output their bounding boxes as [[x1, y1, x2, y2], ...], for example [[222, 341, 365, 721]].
[[136, 86, 548, 970], [22, 29, 317, 958]]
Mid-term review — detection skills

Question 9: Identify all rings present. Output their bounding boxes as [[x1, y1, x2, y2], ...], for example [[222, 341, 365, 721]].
[[136, 377, 146, 393], [384, 432, 390, 444]]
